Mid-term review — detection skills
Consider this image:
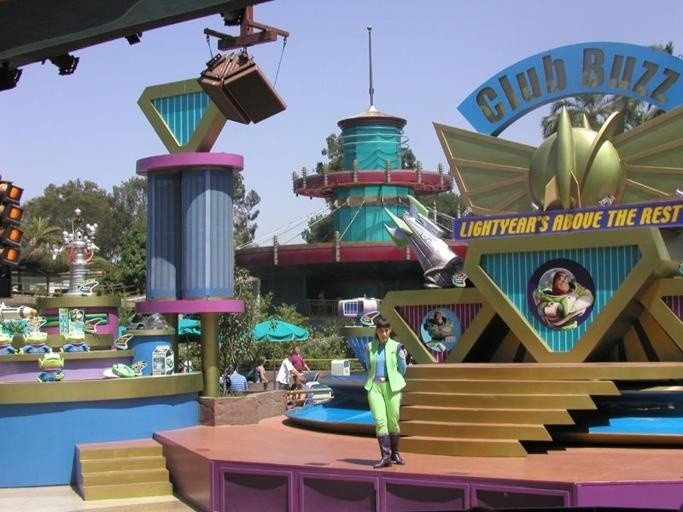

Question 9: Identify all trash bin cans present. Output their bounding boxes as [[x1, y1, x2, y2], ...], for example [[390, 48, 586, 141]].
[[331, 359, 350, 376]]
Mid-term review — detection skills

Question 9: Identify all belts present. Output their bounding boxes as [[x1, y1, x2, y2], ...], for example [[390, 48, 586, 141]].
[[376, 376, 387, 381]]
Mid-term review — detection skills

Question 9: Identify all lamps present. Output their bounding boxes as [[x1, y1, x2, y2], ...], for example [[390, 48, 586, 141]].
[[197, 47, 286, 125], [0, 180, 24, 265]]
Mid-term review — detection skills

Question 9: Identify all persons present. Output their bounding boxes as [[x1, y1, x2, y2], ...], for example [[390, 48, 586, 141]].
[[274, 352, 303, 390], [219, 364, 249, 391], [289, 347, 311, 390], [362, 319, 407, 469], [253, 356, 269, 384], [290, 375, 313, 407]]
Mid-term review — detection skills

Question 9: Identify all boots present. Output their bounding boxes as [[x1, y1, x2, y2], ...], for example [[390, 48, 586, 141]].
[[389, 432, 405, 465], [373, 432, 391, 467]]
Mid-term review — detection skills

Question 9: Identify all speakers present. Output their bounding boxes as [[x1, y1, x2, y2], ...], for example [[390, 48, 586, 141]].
[[1, 180, 24, 266]]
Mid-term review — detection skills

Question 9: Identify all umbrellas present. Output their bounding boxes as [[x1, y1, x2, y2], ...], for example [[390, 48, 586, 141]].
[[240, 321, 308, 344], [174, 318, 224, 372]]
